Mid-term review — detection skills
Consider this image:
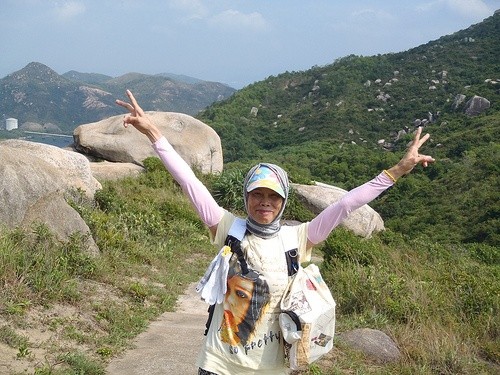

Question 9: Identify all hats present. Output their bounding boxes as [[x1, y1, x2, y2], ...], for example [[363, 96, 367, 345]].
[[246, 166, 286, 199]]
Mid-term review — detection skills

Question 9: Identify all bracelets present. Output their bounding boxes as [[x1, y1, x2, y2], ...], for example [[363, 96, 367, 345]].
[[384, 169, 397, 183]]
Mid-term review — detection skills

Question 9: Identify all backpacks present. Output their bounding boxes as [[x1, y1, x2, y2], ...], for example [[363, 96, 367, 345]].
[[196, 217, 299, 336]]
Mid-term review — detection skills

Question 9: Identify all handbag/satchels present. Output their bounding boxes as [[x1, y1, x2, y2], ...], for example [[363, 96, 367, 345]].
[[279, 264, 336, 370]]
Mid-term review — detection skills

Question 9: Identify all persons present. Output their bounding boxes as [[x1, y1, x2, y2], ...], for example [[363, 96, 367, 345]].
[[219, 262, 270, 346], [114, 88, 437, 375]]
